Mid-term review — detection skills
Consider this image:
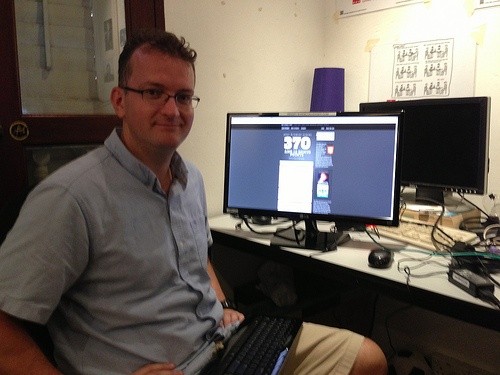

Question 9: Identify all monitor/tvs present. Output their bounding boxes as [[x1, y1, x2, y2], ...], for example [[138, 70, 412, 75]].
[[224, 109, 405, 251], [358, 96, 491, 212]]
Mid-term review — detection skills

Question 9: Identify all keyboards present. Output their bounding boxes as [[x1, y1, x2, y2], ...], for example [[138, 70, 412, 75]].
[[199, 315, 304, 375], [363, 215, 481, 253]]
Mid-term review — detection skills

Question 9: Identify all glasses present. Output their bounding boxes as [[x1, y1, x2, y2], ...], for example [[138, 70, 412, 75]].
[[118, 84, 200, 110]]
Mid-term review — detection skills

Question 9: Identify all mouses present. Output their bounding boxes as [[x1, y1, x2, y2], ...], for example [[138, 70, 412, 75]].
[[369, 248, 394, 266]]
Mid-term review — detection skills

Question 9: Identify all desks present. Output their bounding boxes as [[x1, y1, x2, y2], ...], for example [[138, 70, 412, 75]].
[[207, 213, 500, 330]]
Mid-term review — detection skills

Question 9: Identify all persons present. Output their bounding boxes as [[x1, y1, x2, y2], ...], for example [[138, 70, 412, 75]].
[[0, 29, 389, 375]]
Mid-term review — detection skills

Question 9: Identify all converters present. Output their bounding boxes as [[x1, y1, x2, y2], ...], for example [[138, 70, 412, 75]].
[[448, 269, 494, 297]]
